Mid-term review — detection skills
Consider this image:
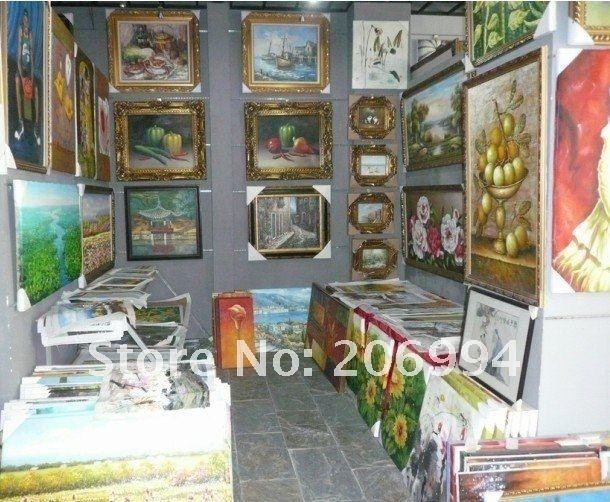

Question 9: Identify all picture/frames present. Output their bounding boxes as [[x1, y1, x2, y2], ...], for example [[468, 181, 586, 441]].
[[1, 2, 117, 315]]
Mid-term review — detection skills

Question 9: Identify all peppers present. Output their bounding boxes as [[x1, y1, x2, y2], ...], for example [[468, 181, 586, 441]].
[[133, 123, 184, 166], [266, 120, 316, 156]]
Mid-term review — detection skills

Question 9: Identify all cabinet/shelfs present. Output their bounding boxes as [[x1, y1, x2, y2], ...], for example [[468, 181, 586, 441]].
[[34, 264, 194, 366]]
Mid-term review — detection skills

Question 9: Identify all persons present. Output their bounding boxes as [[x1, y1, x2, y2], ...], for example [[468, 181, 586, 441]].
[[14, 9, 41, 150]]
